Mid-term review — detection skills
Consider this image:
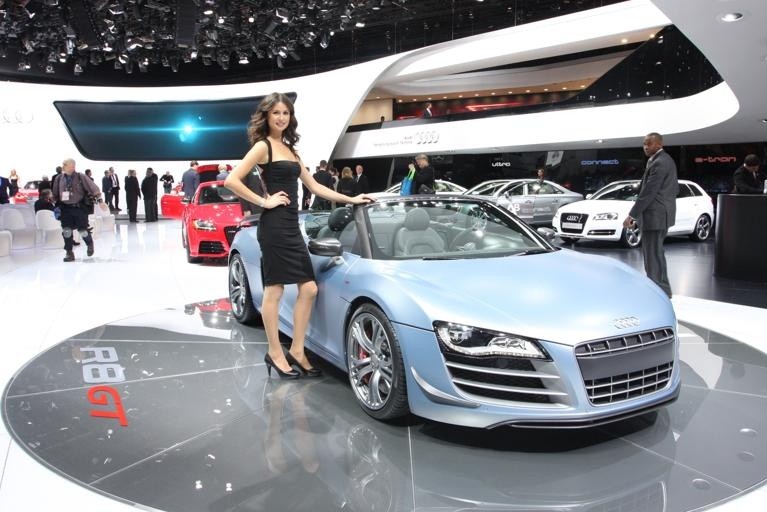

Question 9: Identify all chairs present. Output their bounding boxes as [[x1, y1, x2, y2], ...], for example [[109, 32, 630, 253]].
[[394, 207, 447, 255], [317, 208, 354, 241], [203, 188, 223, 203], [0, 205, 115, 257]]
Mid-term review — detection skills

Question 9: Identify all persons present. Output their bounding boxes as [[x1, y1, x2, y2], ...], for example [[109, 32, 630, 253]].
[[537, 167, 545, 180], [423, 102, 433, 119], [732, 154, 767, 195], [378, 116, 384, 128], [223, 92, 378, 381], [256, 377, 332, 512], [1, 159, 369, 247], [53, 159, 110, 263], [622, 132, 681, 301], [407, 154, 434, 194]]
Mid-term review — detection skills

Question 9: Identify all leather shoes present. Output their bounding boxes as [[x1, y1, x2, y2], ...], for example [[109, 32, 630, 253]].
[[115, 207, 122, 211], [130, 219, 158, 222], [73, 240, 80, 245], [88, 227, 93, 230]]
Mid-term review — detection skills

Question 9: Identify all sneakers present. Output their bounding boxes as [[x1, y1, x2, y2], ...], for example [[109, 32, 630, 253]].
[[64, 253, 75, 261], [87, 243, 94, 255]]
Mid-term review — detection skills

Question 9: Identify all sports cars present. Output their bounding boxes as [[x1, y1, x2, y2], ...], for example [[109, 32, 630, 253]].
[[11, 178, 53, 205], [181, 295, 241, 332], [224, 192, 682, 437], [179, 179, 249, 264], [156, 160, 232, 216], [230, 364, 687, 512]]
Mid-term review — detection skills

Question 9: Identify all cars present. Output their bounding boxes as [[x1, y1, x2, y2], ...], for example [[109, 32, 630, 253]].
[[553, 173, 716, 248], [378, 177, 469, 193], [465, 177, 585, 226]]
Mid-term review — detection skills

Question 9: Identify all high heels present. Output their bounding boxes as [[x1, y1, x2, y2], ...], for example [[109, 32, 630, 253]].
[[286, 352, 321, 377], [264, 353, 300, 379]]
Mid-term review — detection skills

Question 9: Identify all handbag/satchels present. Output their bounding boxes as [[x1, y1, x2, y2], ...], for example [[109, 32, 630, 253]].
[[80, 196, 94, 213], [400, 168, 416, 195], [418, 184, 433, 194]]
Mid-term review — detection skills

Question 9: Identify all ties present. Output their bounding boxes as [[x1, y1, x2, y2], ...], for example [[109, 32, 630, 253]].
[[112, 175, 116, 185]]
